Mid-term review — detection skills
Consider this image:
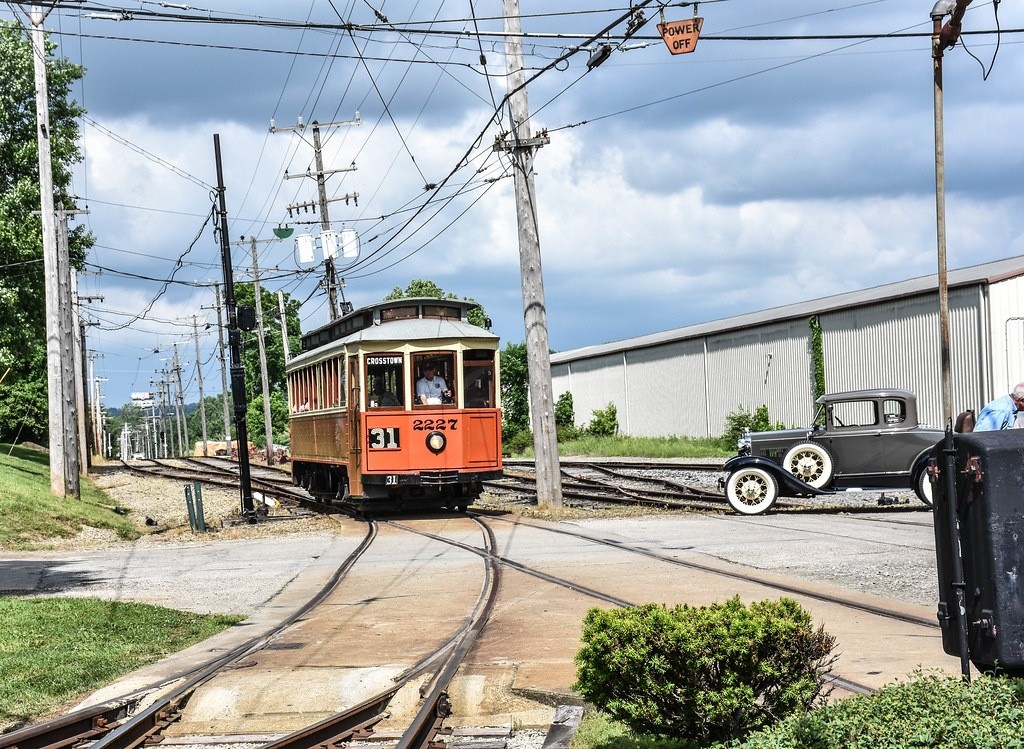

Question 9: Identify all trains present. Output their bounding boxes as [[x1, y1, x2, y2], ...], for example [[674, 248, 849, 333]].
[[288, 295, 505, 514]]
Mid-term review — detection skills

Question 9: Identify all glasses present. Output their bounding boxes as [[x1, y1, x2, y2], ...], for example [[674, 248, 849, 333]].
[[425, 368, 434, 371]]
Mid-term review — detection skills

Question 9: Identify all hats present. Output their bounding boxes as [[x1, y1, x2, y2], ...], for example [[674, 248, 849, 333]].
[[423, 362, 436, 368]]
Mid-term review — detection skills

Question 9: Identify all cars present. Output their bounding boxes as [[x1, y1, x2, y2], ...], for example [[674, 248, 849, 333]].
[[719, 388, 959, 517]]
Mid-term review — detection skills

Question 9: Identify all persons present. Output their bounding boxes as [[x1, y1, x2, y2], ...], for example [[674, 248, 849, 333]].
[[368, 377, 401, 406], [416, 366, 452, 405], [973, 383, 1024, 432]]
